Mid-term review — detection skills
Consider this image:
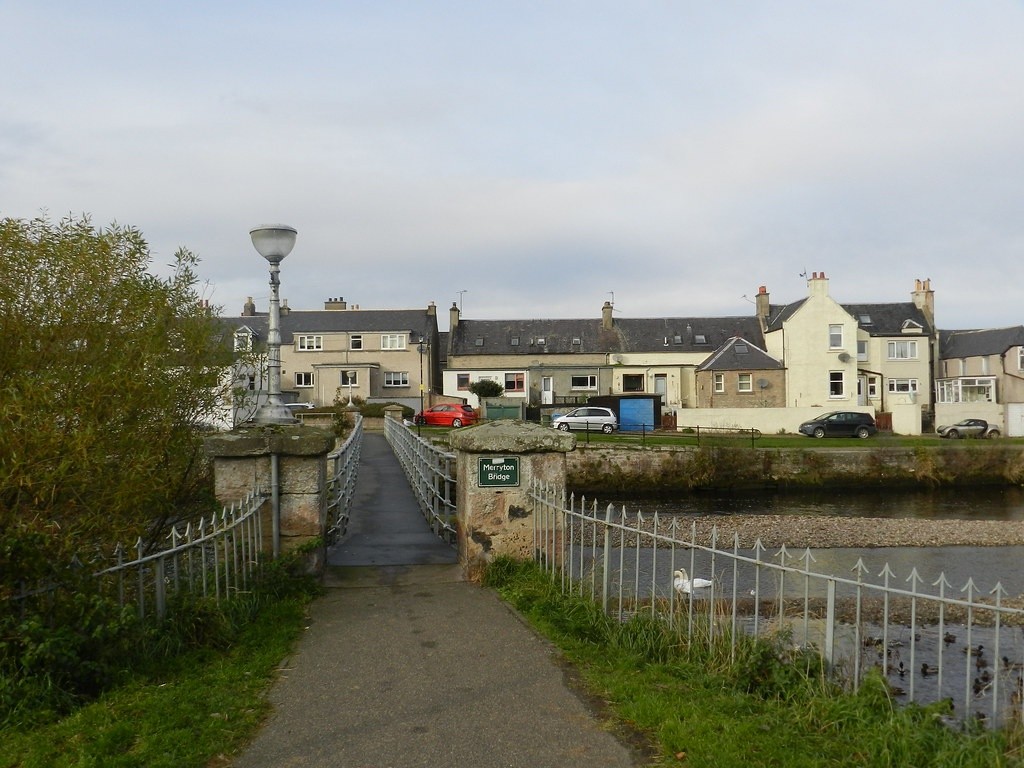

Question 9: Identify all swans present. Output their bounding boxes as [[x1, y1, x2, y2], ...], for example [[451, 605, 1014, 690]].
[[674, 568, 711, 599]]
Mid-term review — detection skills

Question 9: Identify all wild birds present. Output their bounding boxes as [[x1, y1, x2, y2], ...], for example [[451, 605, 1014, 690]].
[[750, 590, 756, 600], [862, 624, 1024, 730], [403, 419, 416, 427]]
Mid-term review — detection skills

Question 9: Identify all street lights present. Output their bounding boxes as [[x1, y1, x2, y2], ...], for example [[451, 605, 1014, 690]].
[[418, 330, 426, 425], [250, 222, 303, 427], [343, 371, 357, 407]]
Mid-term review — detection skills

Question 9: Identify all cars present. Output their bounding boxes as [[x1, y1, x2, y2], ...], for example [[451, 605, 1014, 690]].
[[797, 411, 877, 439], [936, 419, 1001, 440], [552, 406, 618, 434], [415, 403, 479, 429]]
